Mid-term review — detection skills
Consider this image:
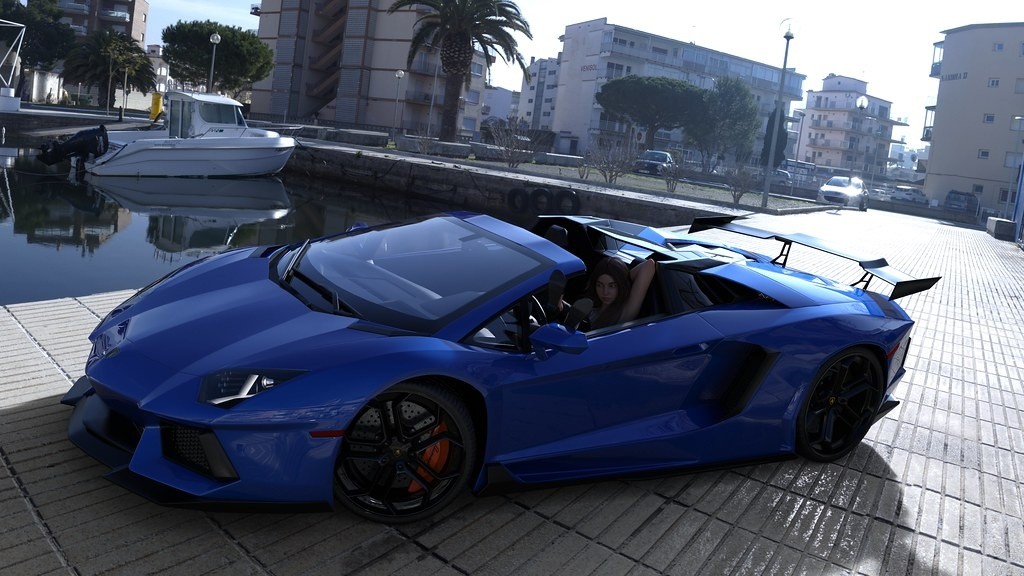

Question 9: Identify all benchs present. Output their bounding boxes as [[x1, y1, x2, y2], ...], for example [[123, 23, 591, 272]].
[[987, 217, 1017, 241]]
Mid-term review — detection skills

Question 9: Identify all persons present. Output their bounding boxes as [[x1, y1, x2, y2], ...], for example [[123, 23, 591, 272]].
[[548, 257, 656, 331]]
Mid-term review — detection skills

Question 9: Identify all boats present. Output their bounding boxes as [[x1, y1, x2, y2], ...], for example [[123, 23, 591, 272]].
[[58, 169, 290, 228], [38, 93, 296, 178], [0, 147, 42, 175]]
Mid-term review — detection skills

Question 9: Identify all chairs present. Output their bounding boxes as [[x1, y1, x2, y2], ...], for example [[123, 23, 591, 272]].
[[544, 224, 714, 322]]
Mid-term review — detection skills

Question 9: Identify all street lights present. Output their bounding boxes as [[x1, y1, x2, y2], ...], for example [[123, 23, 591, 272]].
[[208, 32, 221, 92], [392, 70, 405, 142], [761, 18, 803, 208], [844, 96, 869, 206]]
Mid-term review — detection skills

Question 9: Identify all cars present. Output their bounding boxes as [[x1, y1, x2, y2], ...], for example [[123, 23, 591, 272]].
[[817, 176, 870, 212], [634, 149, 676, 175]]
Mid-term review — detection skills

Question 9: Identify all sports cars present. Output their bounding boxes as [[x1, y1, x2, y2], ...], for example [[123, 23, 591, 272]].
[[59, 213, 944, 518]]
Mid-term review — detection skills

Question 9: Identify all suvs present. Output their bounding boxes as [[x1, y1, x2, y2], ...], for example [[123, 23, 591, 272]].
[[891, 186, 929, 206], [944, 189, 980, 215], [752, 169, 791, 188]]
